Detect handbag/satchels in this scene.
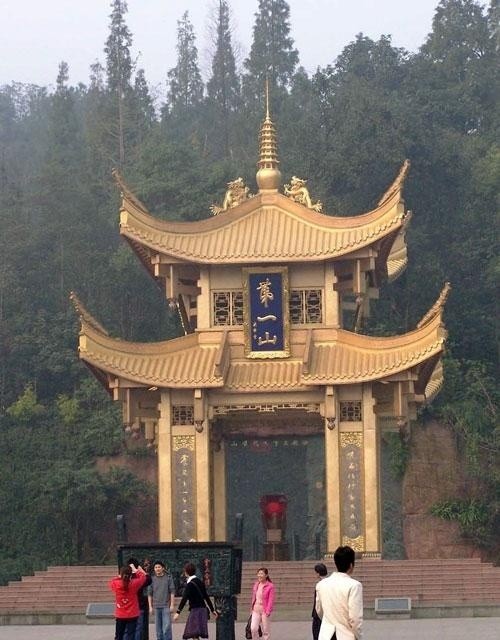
[206,607,211,620]
[245,615,262,639]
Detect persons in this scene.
[314,546,364,640]
[109,563,146,640]
[172,563,218,640]
[146,560,176,640]
[119,557,152,640]
[311,562,336,640]
[249,567,276,640]
